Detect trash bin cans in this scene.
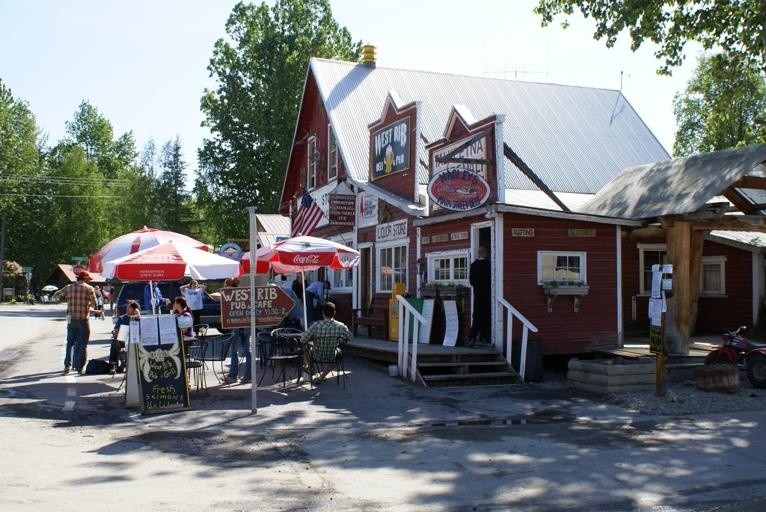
[512,340,544,383]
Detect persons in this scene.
[51,272,207,378]
[281,273,331,331]
[300,300,352,383]
[464,247,490,347]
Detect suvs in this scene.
[111,280,221,333]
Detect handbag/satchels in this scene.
[86,359,110,374]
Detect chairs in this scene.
[113,322,350,397]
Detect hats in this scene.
[79,272,93,280]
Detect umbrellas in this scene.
[88,225,210,273]
[100,240,243,314]
[240,232,362,331]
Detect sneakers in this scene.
[313,378,321,385]
[221,375,237,383]
[304,364,315,375]
[239,375,251,382]
[109,365,118,374]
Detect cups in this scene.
[385,145,394,173]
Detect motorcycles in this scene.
[704,325,766,391]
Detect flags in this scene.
[292,187,322,236]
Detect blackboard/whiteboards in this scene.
[134,327,192,416]
